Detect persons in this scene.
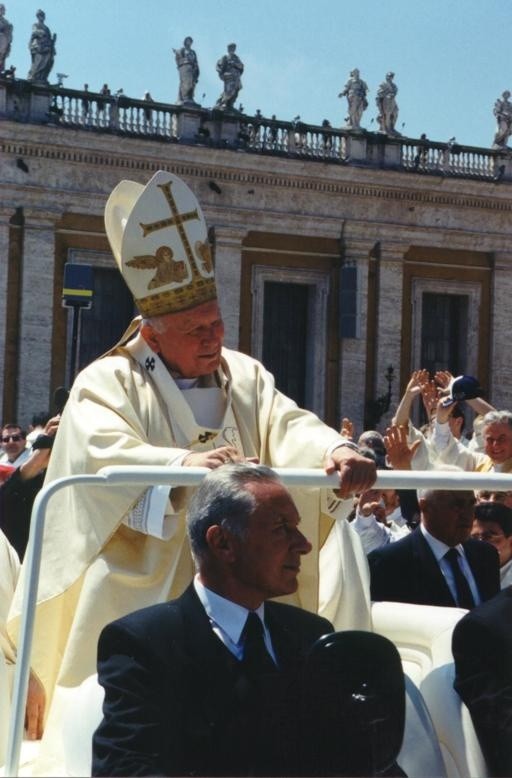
[1,4,14,72]
[142,91,156,135]
[1,365,512,611]
[416,134,430,169]
[28,9,58,82]
[375,71,400,131]
[173,36,200,103]
[2,300,379,778]
[338,68,369,126]
[95,84,111,128]
[80,83,91,122]
[492,90,512,149]
[268,114,279,150]
[449,582,511,778]
[252,109,263,142]
[58,256,378,644]
[216,42,245,108]
[87,458,414,777]
[320,119,333,157]
[0,528,54,745]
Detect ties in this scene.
[444,548,475,610]
[243,612,275,676]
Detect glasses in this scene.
[3,435,20,442]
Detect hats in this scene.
[442,375,482,407]
[104,170,217,318]
